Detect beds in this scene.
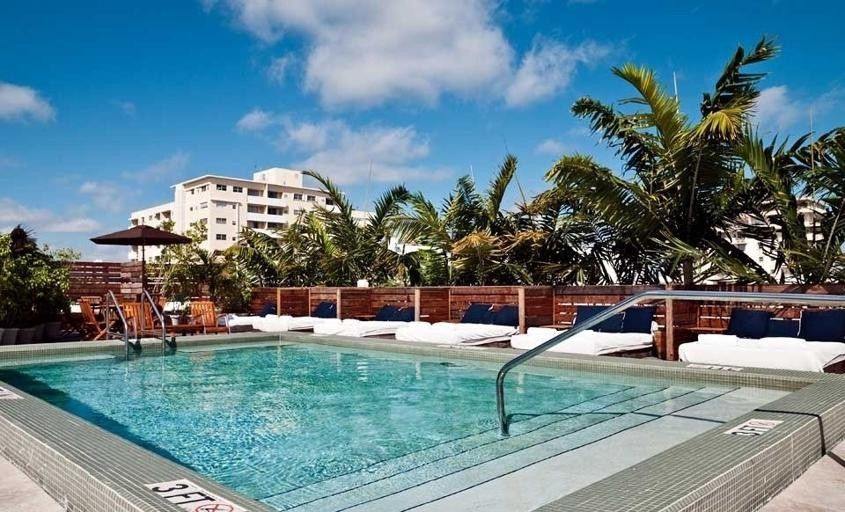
[678,335,845,374]
[511,316,658,358]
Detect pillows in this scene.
[261,304,277,317]
[573,305,657,333]
[375,304,414,322]
[311,302,336,318]
[727,308,845,341]
[460,304,519,326]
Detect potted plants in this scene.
[0,225,81,345]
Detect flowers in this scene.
[163,301,190,315]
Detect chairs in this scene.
[313,320,414,337]
[225,314,278,333]
[122,302,160,338]
[79,302,119,340]
[259,316,337,331]
[395,323,520,347]
[191,301,229,335]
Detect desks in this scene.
[155,323,204,336]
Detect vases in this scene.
[170,315,180,325]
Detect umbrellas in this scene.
[88,223,194,297]
